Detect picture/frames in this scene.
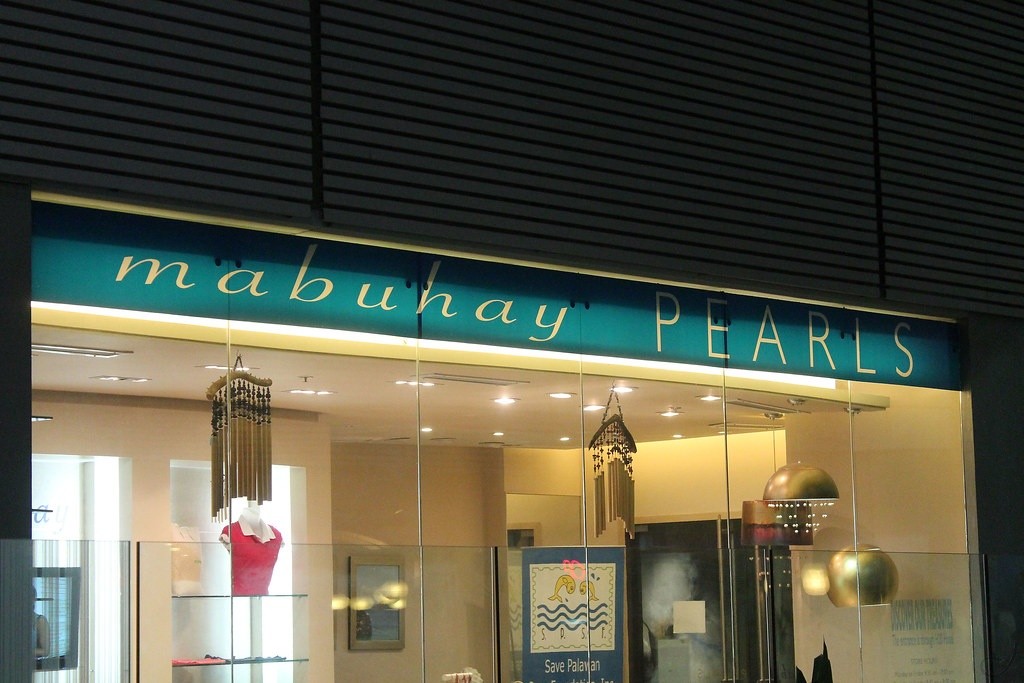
[506,523,543,557]
[350,555,405,650]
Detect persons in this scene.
[220,507,285,596]
[33,585,50,657]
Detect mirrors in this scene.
[506,492,581,683]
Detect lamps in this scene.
[740,398,899,608]
